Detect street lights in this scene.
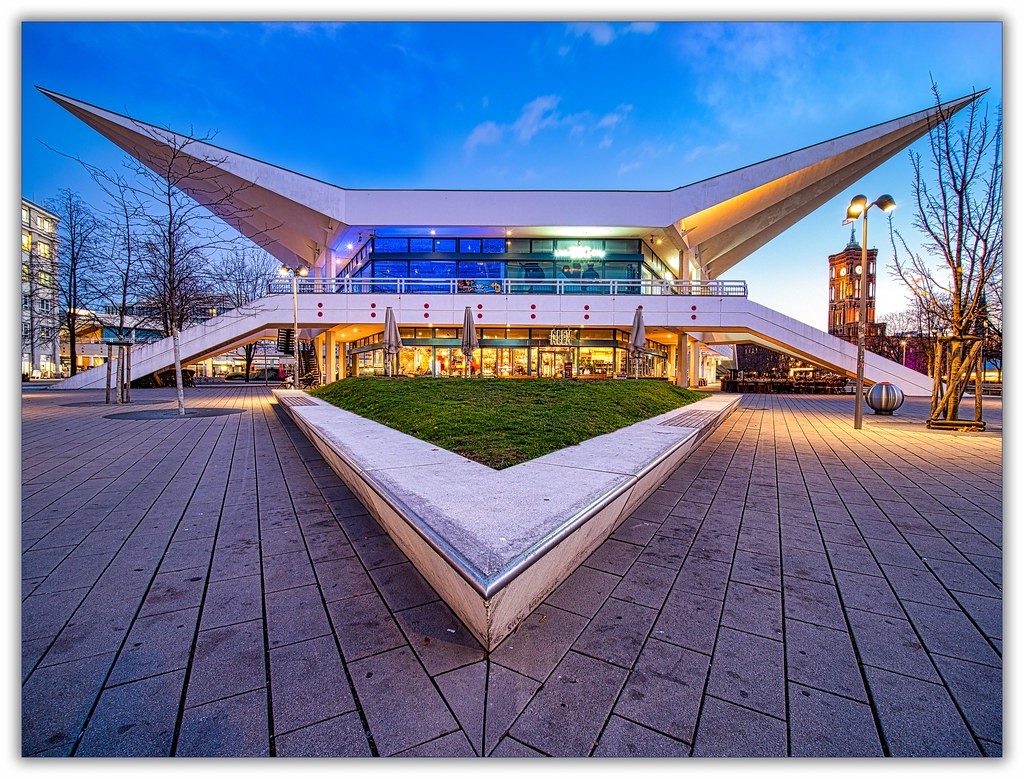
[281,264,309,390]
[846,194,897,428]
[901,341,906,366]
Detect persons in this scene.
[582,262,600,291]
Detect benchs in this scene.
[966,384,1002,396]
[277,382,294,389]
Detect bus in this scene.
[254,369,279,380]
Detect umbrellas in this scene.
[382,307,403,376]
[461,307,478,380]
[631,308,646,380]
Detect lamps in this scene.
[650,235,654,244]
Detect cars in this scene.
[225,373,246,380]
